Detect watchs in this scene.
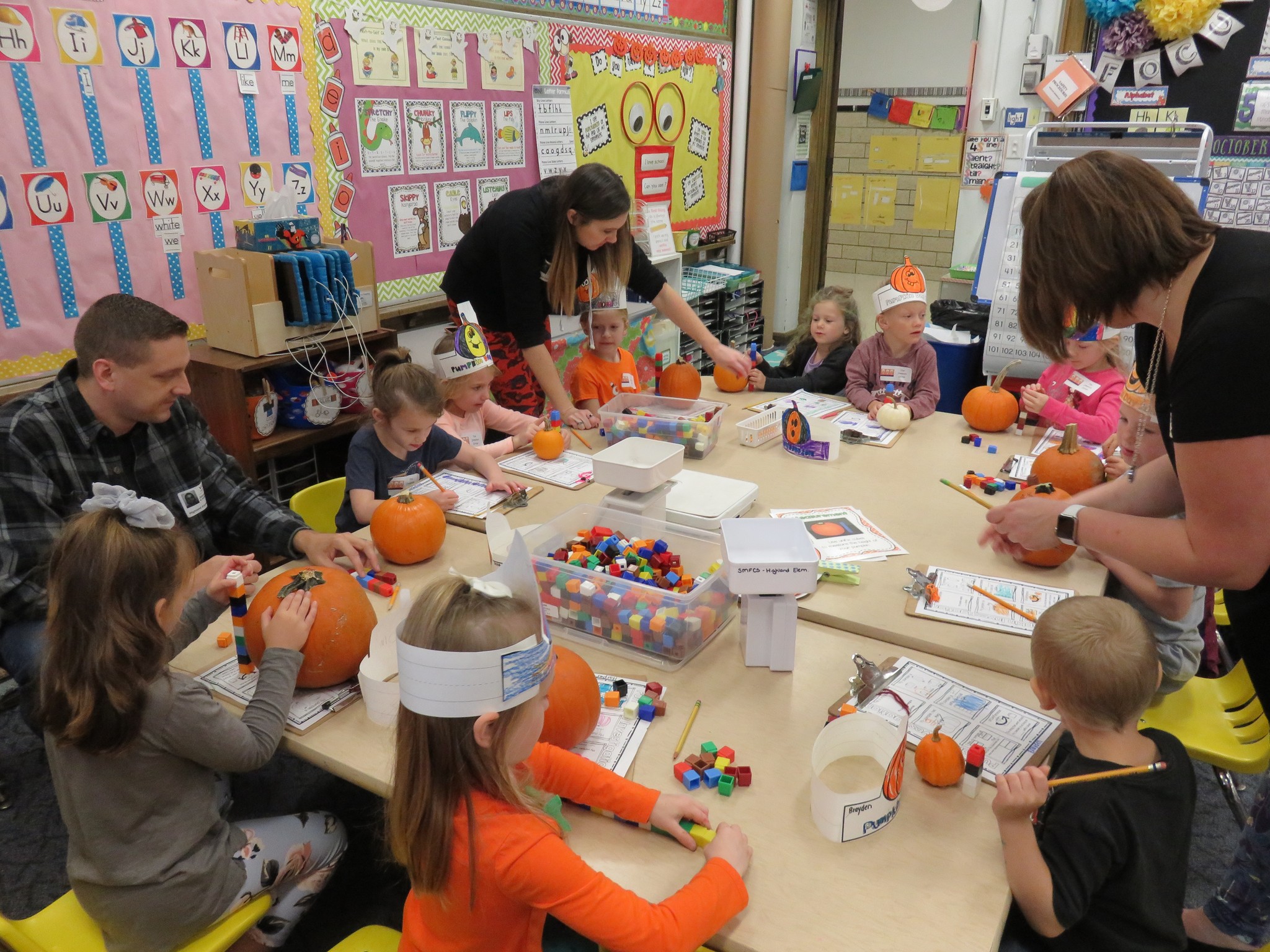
[1055,504,1086,547]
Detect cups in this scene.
[683,230,700,249]
[672,231,688,251]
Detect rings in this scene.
[577,421,583,426]
[743,354,746,358]
[588,414,593,419]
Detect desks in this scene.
[169,522,1061,952]
[391,376,1111,680]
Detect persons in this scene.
[1085,358,1219,683]
[434,330,571,472]
[0,295,380,742]
[439,163,751,430]
[745,285,862,395]
[38,482,348,952]
[1019,303,1126,444]
[992,596,1197,952]
[846,277,940,421]
[978,151,1270,952]
[334,345,527,533]
[571,309,655,420]
[389,575,752,952]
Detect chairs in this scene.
[1138,657,1269,774]
[0,890,271,952]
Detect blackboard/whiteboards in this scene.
[971,171,1211,332]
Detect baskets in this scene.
[681,277,708,302]
[707,228,738,243]
[681,266,730,294]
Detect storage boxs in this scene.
[509,504,819,672]
[592,392,728,492]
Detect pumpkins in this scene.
[370,490,447,564]
[962,358,1022,432]
[244,565,379,688]
[876,396,910,430]
[1029,422,1106,495]
[915,724,965,786]
[659,357,701,409]
[537,644,602,751]
[713,340,749,392]
[1006,482,1080,567]
[533,417,564,459]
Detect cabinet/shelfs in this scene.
[185,328,398,491]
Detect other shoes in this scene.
[1181,905,1259,952]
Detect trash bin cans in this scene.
[921,297,992,415]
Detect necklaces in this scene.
[1127,277,1175,484]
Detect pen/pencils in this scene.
[742,398,778,410]
[417,463,447,492]
[1047,762,1168,787]
[673,699,702,761]
[570,428,592,450]
[745,424,778,443]
[388,582,401,610]
[966,582,1039,623]
[937,479,994,510]
